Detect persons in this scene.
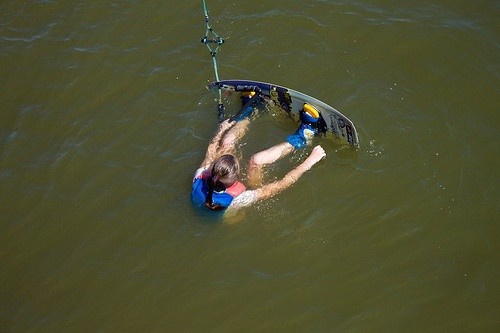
[190,95,326,225]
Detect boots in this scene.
[284,104,319,151]
[232,91,268,124]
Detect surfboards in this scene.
[206,79,359,144]
